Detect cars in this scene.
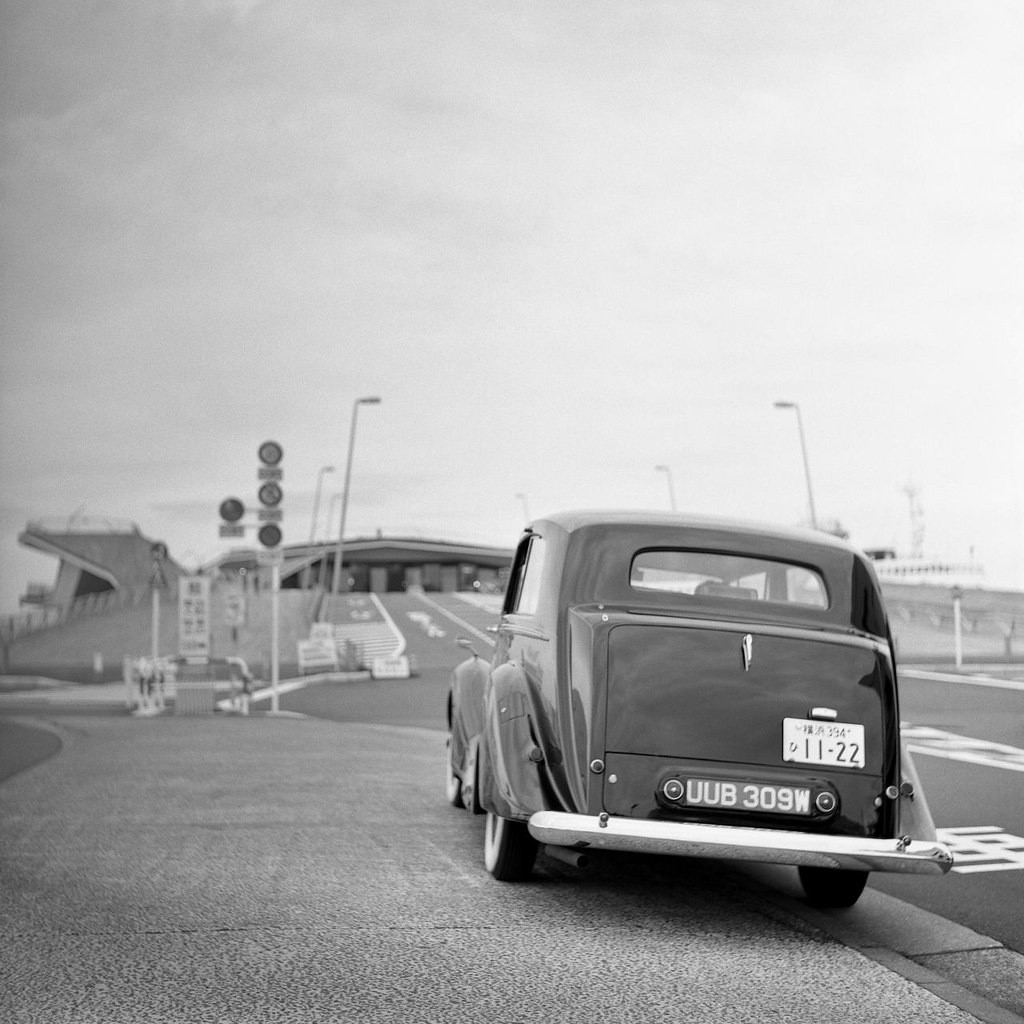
[445,506,956,913]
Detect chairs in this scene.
[700,582,757,600]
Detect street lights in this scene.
[774,398,816,532]
[655,465,675,512]
[325,393,383,641]
[298,465,336,617]
